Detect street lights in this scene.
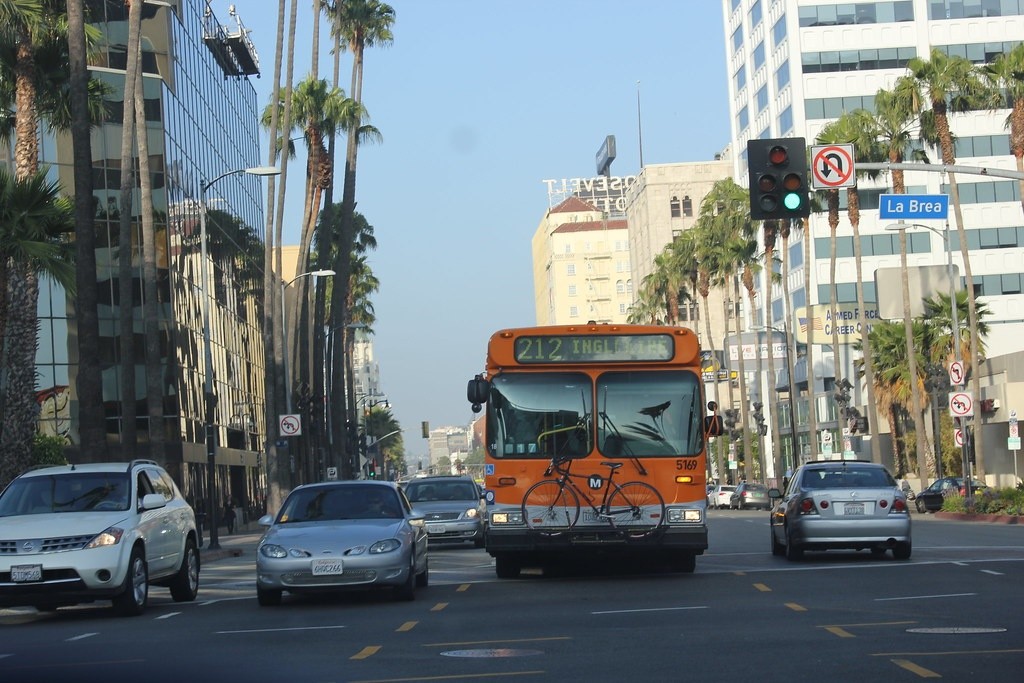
[749,324,798,470]
[923,363,946,478]
[354,392,386,474]
[886,221,972,510]
[726,410,740,486]
[279,269,338,500]
[368,399,391,480]
[834,377,869,460]
[204,166,283,548]
[753,402,768,486]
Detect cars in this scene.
[767,460,912,562]
[730,482,772,512]
[406,476,486,547]
[915,476,992,514]
[707,485,715,495]
[707,482,738,510]
[255,479,428,603]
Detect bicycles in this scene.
[521,455,665,539]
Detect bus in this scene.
[467,321,725,578]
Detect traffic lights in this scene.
[457,461,461,471]
[370,464,374,477]
[746,136,809,220]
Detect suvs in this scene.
[0,458,203,613]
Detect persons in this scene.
[449,486,469,499]
[223,508,236,534]
[101,479,143,509]
[707,476,747,485]
[418,486,438,501]
[362,493,389,514]
